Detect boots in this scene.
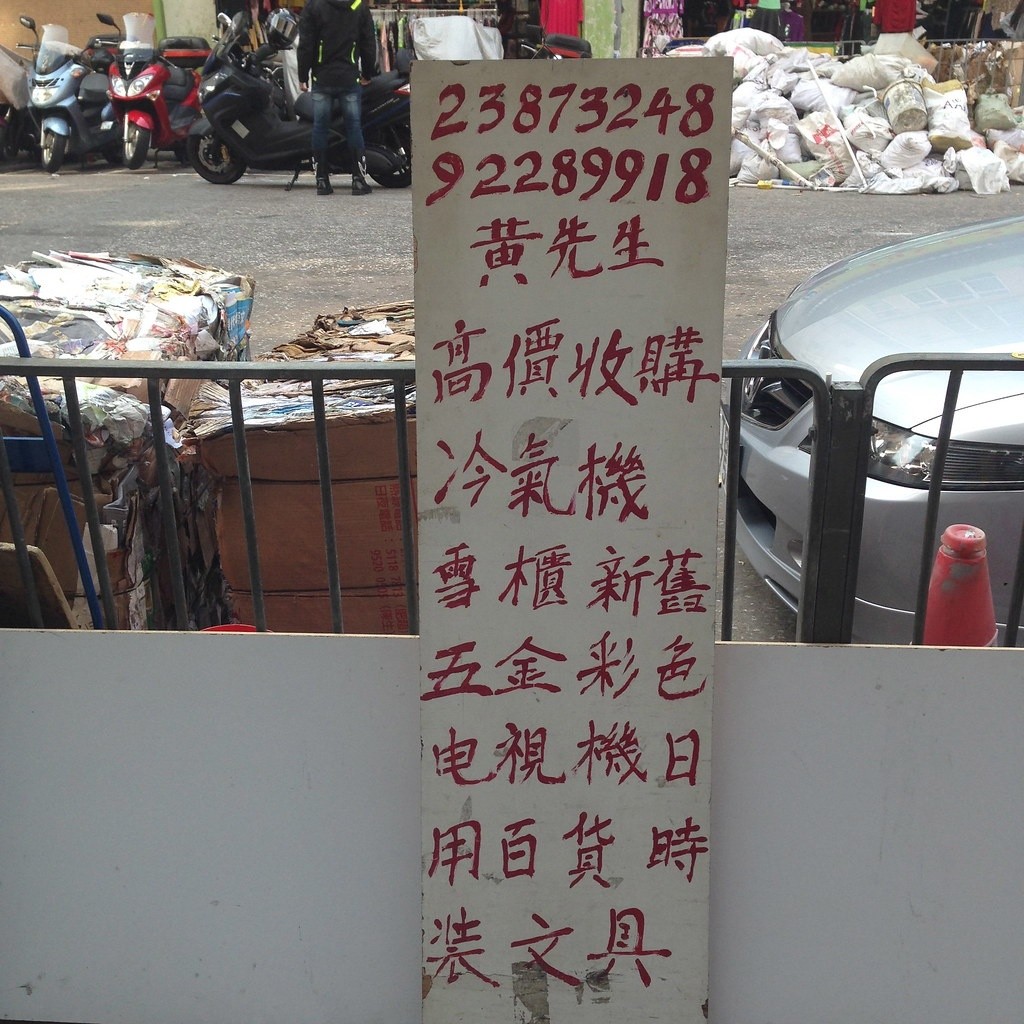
[348,148,371,194]
[312,147,335,195]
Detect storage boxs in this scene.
[162,38,211,71]
[86,32,121,65]
[204,412,418,635]
[542,34,594,59]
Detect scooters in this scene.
[519,23,595,59]
[180,9,414,192]
[0,12,214,175]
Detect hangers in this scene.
[782,2,792,12]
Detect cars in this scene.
[722,214,1024,647]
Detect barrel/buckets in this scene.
[882,77,927,134]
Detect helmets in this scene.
[91,48,116,73]
[266,8,299,48]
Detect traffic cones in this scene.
[929,525,1002,653]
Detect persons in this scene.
[495,0,515,59]
[297,0,377,196]
[231,0,268,31]
[262,0,300,100]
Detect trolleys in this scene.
[1,304,104,630]
